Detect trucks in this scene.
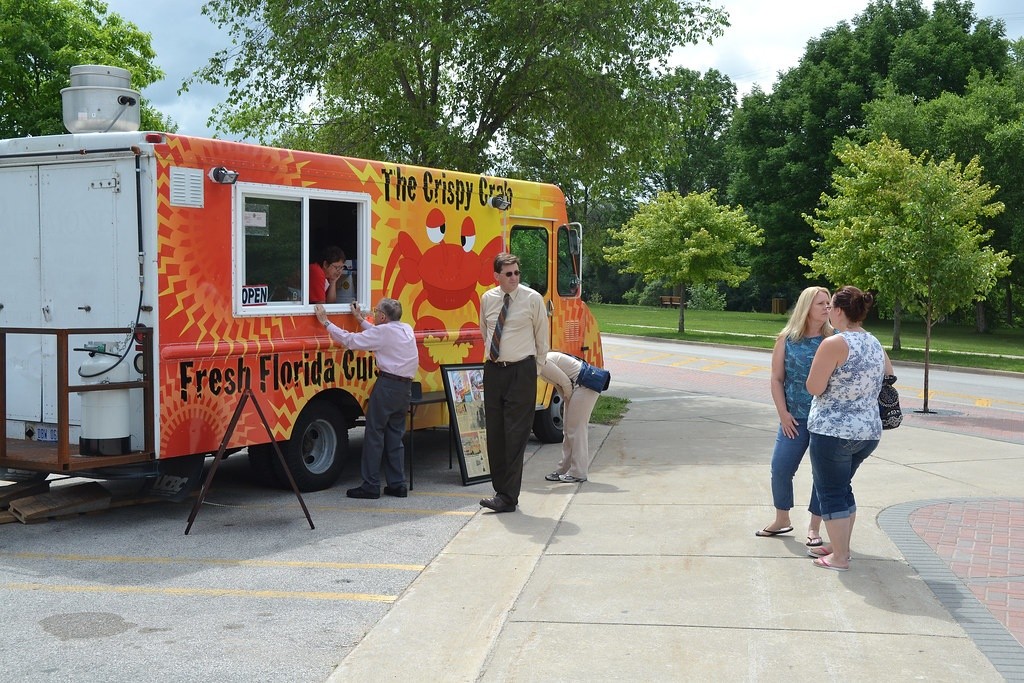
[0,130,605,494]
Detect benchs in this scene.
[670,297,688,310]
[660,295,678,308]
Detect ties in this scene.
[490,295,510,363]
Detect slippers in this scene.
[805,536,823,546]
[812,556,850,572]
[755,524,793,536]
[807,547,852,560]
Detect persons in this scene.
[805,285,895,572]
[314,297,419,499]
[534,347,601,483]
[755,286,840,546]
[478,252,550,510]
[294,244,346,303]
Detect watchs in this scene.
[324,322,329,326]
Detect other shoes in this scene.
[479,495,516,513]
[383,485,410,498]
[347,486,380,499]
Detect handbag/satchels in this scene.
[878,346,904,429]
[575,360,611,393]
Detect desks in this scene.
[407,390,452,491]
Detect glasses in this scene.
[332,262,346,272]
[826,303,844,314]
[500,269,521,277]
[372,307,387,319]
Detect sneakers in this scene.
[558,473,588,482]
[545,472,560,481]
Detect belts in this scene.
[485,357,532,368]
